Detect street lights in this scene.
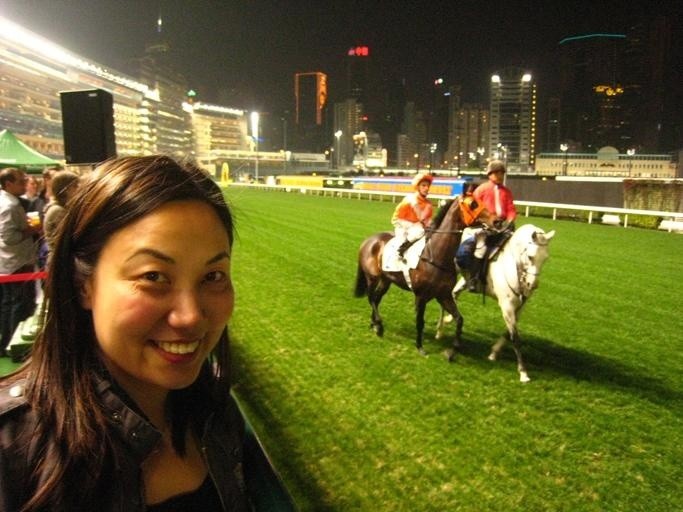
[496,144,509,189]
[360,132,368,169]
[625,147,635,178]
[559,143,567,176]
[334,129,341,168]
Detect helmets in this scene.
[486,160,505,176]
[412,173,433,189]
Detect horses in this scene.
[435,221,557,385]
[352,182,506,362]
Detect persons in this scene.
[0,165,80,359]
[0,149,237,511]
[388,173,435,266]
[467,160,517,292]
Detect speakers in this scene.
[58,88,117,166]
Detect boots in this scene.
[463,271,476,291]
[396,237,412,259]
